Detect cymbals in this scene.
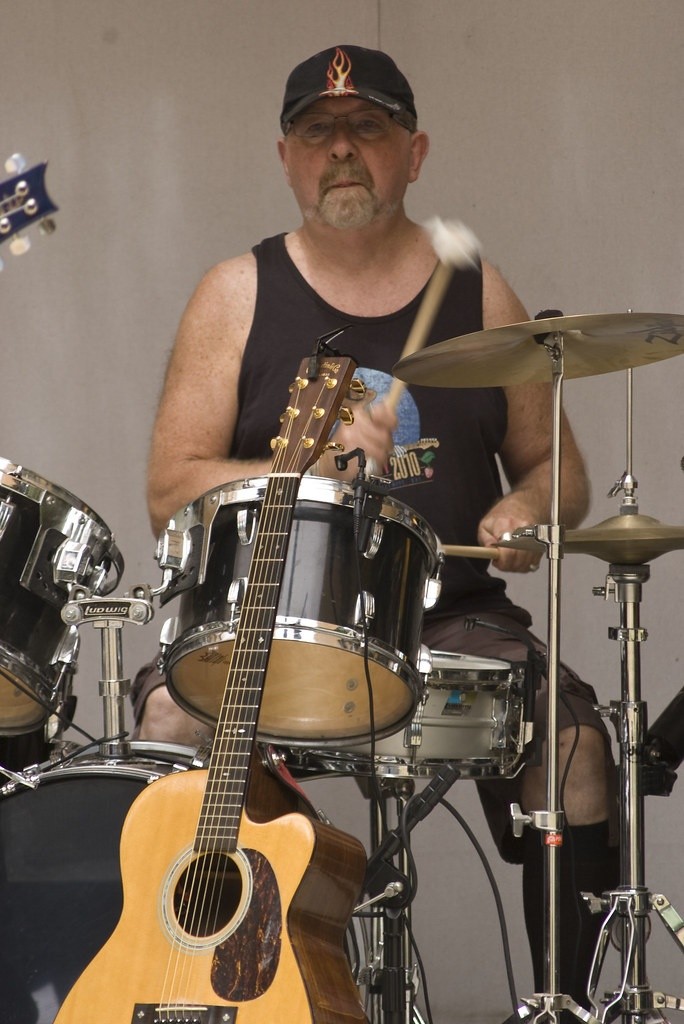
[392,310,684,389]
[491,514,684,564]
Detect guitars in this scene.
[55,348,369,1022]
[0,153,57,267]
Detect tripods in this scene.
[494,333,683,1024]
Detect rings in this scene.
[530,564,539,571]
[503,532,513,541]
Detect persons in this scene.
[130,44,623,1024]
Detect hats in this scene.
[280,45,418,131]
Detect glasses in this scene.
[284,107,413,138]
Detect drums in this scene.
[273,647,528,779]
[162,473,445,748]
[0,459,118,740]
[0,738,369,1024]
